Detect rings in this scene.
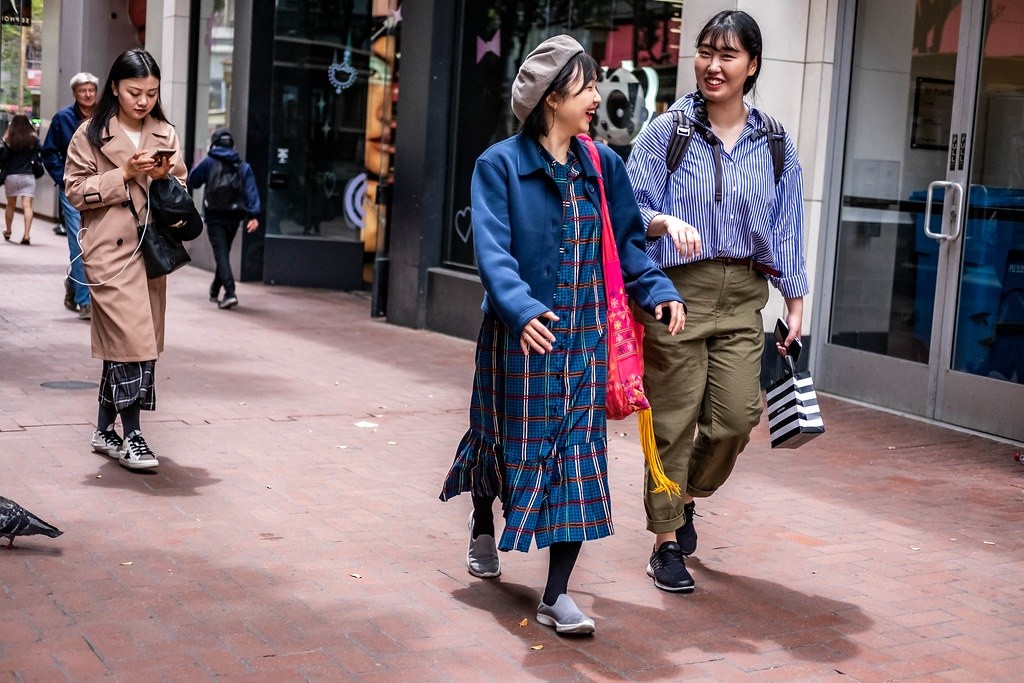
[523,334,529,340]
[682,313,686,317]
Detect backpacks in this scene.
[210,154,246,218]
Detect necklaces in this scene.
[558,156,567,163]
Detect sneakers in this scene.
[92,430,124,459]
[120,431,159,468]
[678,502,696,556]
[647,540,695,591]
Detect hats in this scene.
[511,34,583,123]
[70,71,101,86]
[212,128,235,148]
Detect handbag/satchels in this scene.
[766,355,826,449]
[137,223,192,278]
[32,156,45,178]
[148,173,204,240]
[606,302,650,421]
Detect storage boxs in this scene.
[910,186,1024,376]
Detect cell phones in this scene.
[774,318,802,364]
[149,149,176,167]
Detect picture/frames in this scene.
[911,77,954,150]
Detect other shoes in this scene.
[468,512,501,578]
[536,594,595,634]
[54,227,67,235]
[220,294,238,309]
[211,293,218,300]
[79,305,93,319]
[20,237,31,244]
[4,231,11,240]
[64,278,77,311]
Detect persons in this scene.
[1,112,42,245]
[626,10,809,591]
[439,34,688,633]
[187,129,260,310]
[64,49,187,469]
[303,171,325,235]
[42,71,99,318]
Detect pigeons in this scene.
[0,496,64,551]
